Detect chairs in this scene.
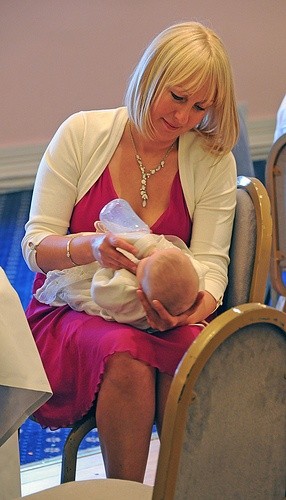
[21,303,286,500]
[265,133,285,312]
[60,176,272,483]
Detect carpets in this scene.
[1,160,286,473]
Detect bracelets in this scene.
[66,232,81,267]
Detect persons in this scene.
[22,22,237,484]
[45,232,210,330]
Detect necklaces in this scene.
[128,118,177,208]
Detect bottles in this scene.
[99,199,152,246]
